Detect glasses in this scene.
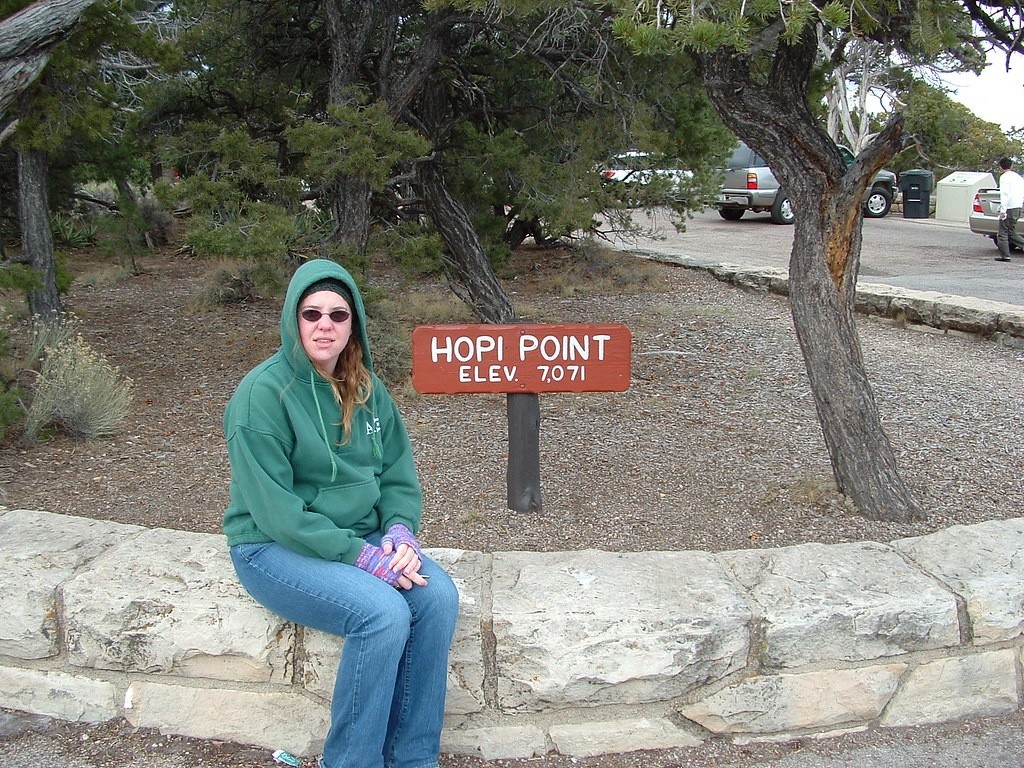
[298,310,353,322]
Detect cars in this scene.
[590,151,698,208]
[969,188,1024,251]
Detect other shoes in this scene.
[995,257,1010,262]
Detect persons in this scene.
[143,167,195,218]
[222,259,459,768]
[995,156,1024,262]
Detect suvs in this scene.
[702,140,899,225]
